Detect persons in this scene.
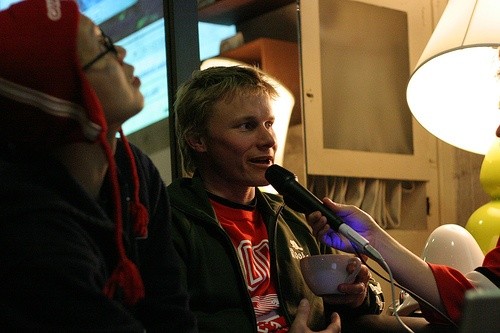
[0,0,198,333]
[166,66,385,333]
[305,197,500,333]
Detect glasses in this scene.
[82,29,118,72]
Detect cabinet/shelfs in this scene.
[162,0,443,318]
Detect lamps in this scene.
[406,0,500,156]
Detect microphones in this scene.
[264,165,369,250]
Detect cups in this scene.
[300,255,362,296]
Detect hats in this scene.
[0,0,148,303]
